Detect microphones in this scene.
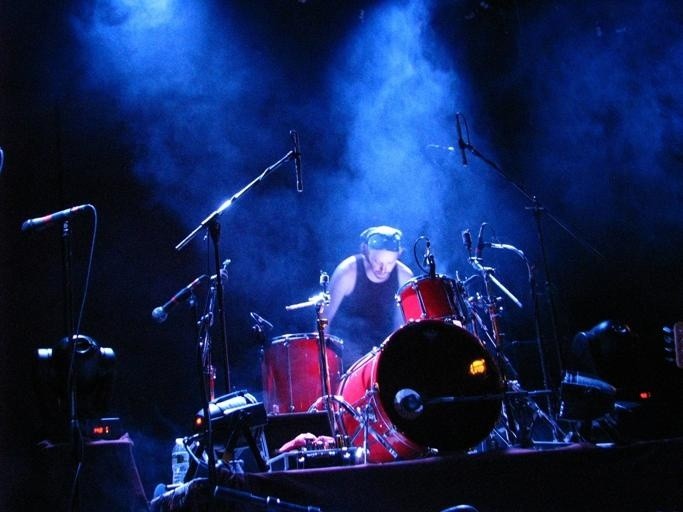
[252,313,274,330]
[21,204,89,236]
[293,133,303,192]
[459,274,480,295]
[475,226,483,261]
[423,241,430,273]
[455,114,469,165]
[462,229,473,247]
[403,392,423,412]
[150,274,208,324]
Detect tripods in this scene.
[461,257,579,443]
[305,299,402,462]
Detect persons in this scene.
[318,225,414,373]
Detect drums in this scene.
[395,273,469,327]
[333,320,503,462]
[259,332,344,412]
